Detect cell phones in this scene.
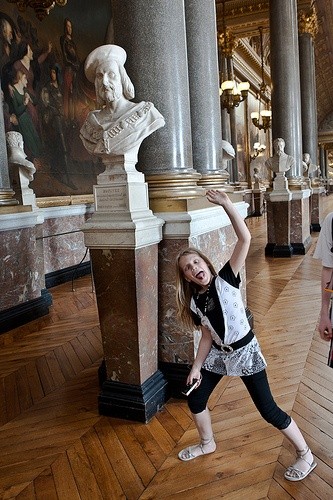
[182,379,199,396]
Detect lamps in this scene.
[250,27,272,133]
[216,0,250,114]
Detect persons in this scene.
[6,132,36,182]
[265,138,293,173]
[303,153,317,173]
[313,211,333,370]
[79,45,165,156]
[176,190,317,481]
